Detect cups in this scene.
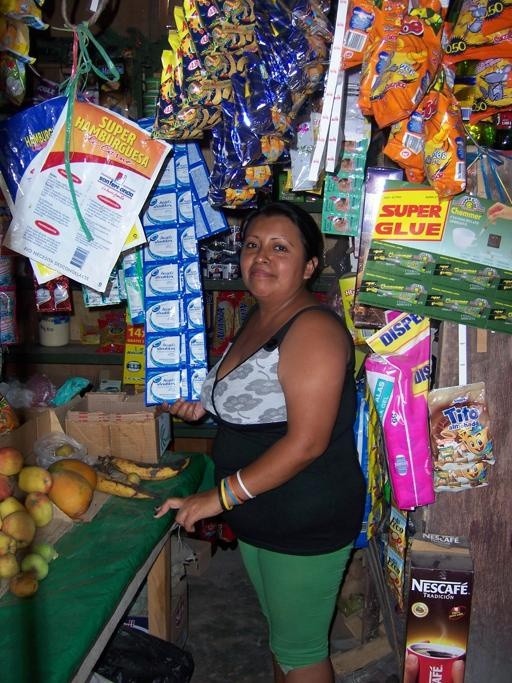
[408,643,464,683]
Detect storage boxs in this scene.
[0,390,172,500]
[172,535,213,577]
[120,578,189,651]
[309,213,339,273]
[69,290,113,344]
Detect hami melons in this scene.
[48,459,97,491]
[48,469,92,518]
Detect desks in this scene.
[1,340,135,396]
[167,421,219,455]
[0,447,217,682]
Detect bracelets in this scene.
[216,468,256,514]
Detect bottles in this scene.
[37,314,71,347]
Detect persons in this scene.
[152,201,364,682]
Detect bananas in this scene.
[97,476,158,498]
[108,456,191,481]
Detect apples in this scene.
[0,447,53,598]
